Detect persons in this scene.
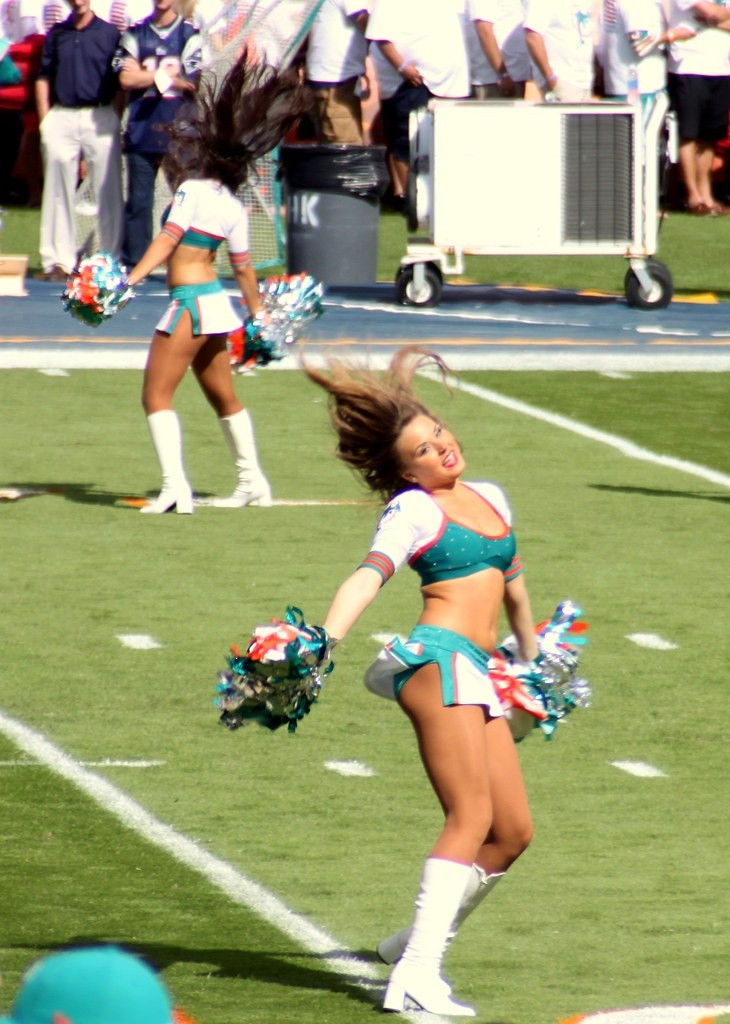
[364,0,472,213]
[303,0,372,148]
[112,0,203,285]
[467,0,532,101]
[313,408,542,1017]
[601,1,696,222]
[666,0,730,218]
[122,151,270,516]
[522,0,602,101]
[34,0,127,282]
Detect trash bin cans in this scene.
[274,141,391,288]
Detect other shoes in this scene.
[41,265,69,282]
[689,200,726,221]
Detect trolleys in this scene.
[396,91,676,312]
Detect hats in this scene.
[3,946,172,1022]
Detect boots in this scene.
[213,408,273,508]
[380,857,506,1018]
[140,411,193,515]
